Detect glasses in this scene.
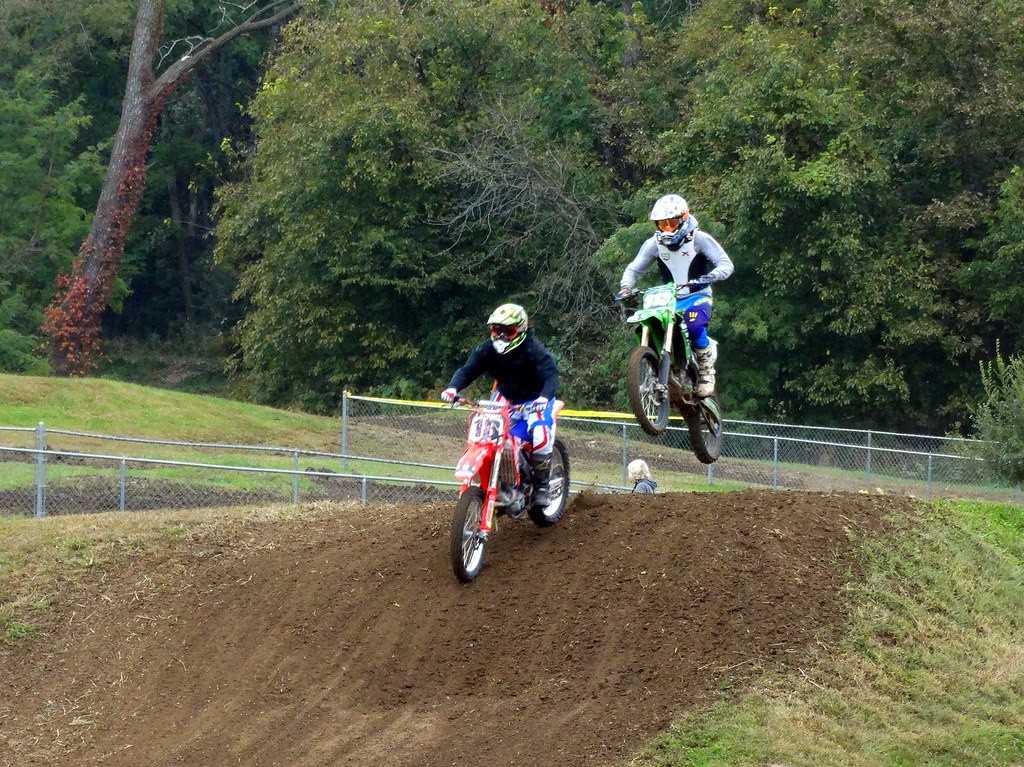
[658,220,680,233]
[491,325,518,339]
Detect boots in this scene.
[532,459,552,508]
[695,342,716,395]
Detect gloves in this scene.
[441,388,456,403]
[699,275,712,284]
[618,289,628,298]
[530,397,548,412]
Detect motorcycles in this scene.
[617,277,724,464]
[442,392,571,581]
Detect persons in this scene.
[441,303,559,508]
[628,460,657,494]
[618,194,734,397]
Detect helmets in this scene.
[649,193,699,245]
[486,303,528,354]
